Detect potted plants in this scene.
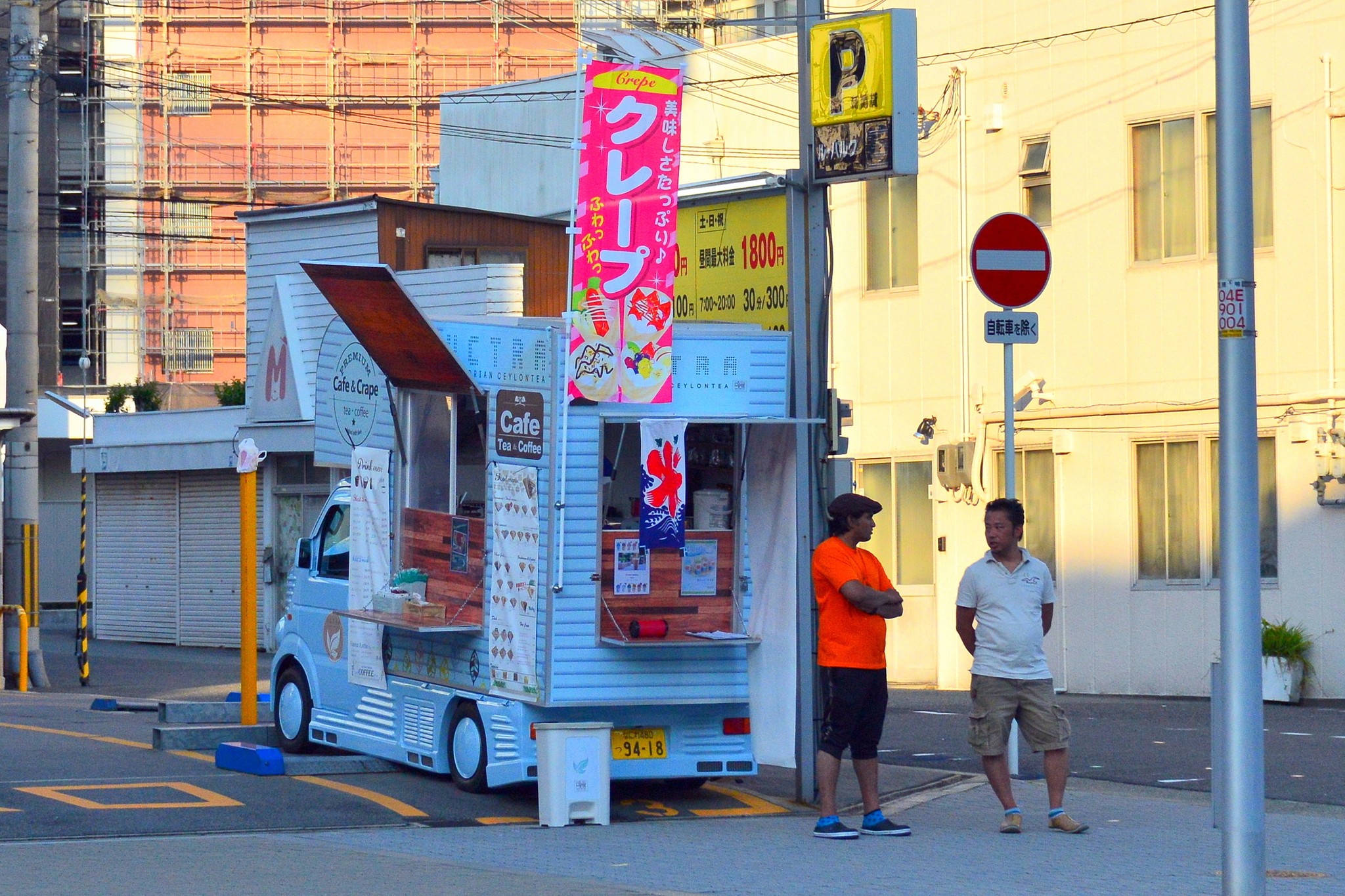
[1261,614,1337,704]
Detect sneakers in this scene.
[1000,813,1023,833]
[1048,813,1089,834]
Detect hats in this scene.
[827,492,883,514]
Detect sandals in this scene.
[860,817,912,837]
[813,822,859,840]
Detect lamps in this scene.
[913,415,937,440]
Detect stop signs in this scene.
[969,210,1054,306]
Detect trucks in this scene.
[270,310,799,791]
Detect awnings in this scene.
[602,419,828,528]
[299,259,487,464]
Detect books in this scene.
[685,630,748,640]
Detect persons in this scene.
[811,492,913,839]
[955,498,1089,835]
